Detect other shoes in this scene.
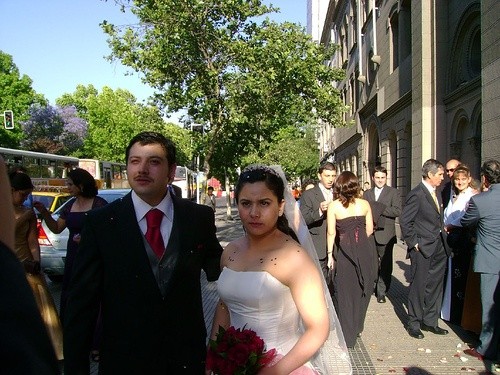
[464,349,486,357]
[375,293,386,303]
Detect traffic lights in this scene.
[4,110,14,129]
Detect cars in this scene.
[23,188,132,283]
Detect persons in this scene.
[399,158,449,339]
[324,171,376,350]
[202,186,216,216]
[300,161,340,301]
[62,130,224,375]
[361,166,402,303]
[288,177,318,242]
[443,156,500,365]
[359,181,372,198]
[0,151,115,375]
[202,165,332,375]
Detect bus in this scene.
[0,147,80,193]
[172,165,208,199]
[61,158,131,189]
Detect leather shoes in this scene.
[421,323,449,335]
[404,324,425,339]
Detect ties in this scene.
[432,192,440,214]
[325,190,331,206]
[143,207,165,258]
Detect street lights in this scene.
[183,106,193,169]
